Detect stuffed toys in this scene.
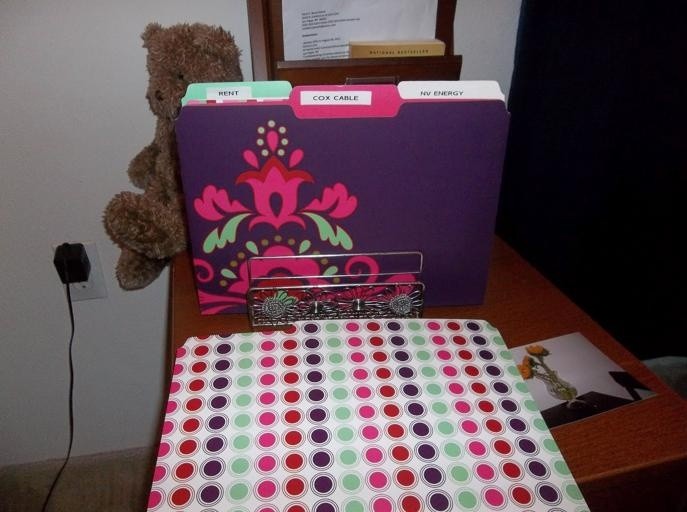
[101,21,245,291]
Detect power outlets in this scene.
[52,242,107,304]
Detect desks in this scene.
[167,231,685,511]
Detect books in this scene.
[347,39,446,58]
